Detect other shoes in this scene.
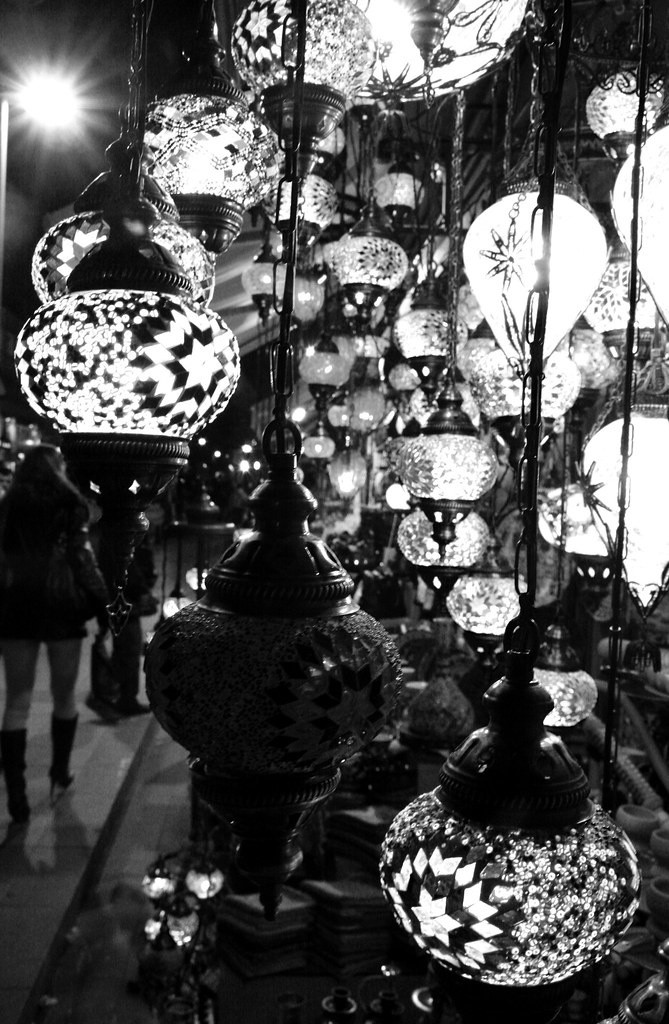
[115,697,148,714]
[85,693,121,722]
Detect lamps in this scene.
[15,0,669,1024]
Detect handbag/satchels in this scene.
[47,530,109,620]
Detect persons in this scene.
[86,532,160,720]
[0,444,110,823]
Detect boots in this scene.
[1,729,29,821]
[48,713,78,799]
